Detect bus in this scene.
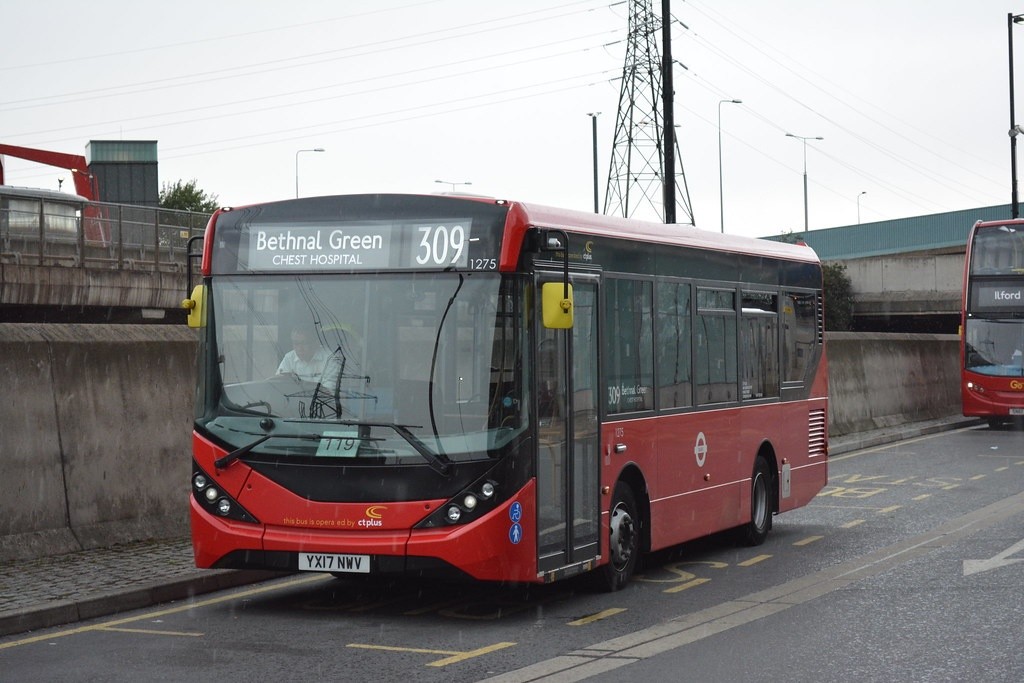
[179,192,832,594]
[957,218,1024,431]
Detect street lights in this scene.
[717,99,743,234]
[71,167,95,201]
[857,191,867,224]
[587,111,601,214]
[784,133,824,231]
[434,180,473,193]
[1005,12,1024,219]
[295,147,327,198]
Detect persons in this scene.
[274,321,343,419]
